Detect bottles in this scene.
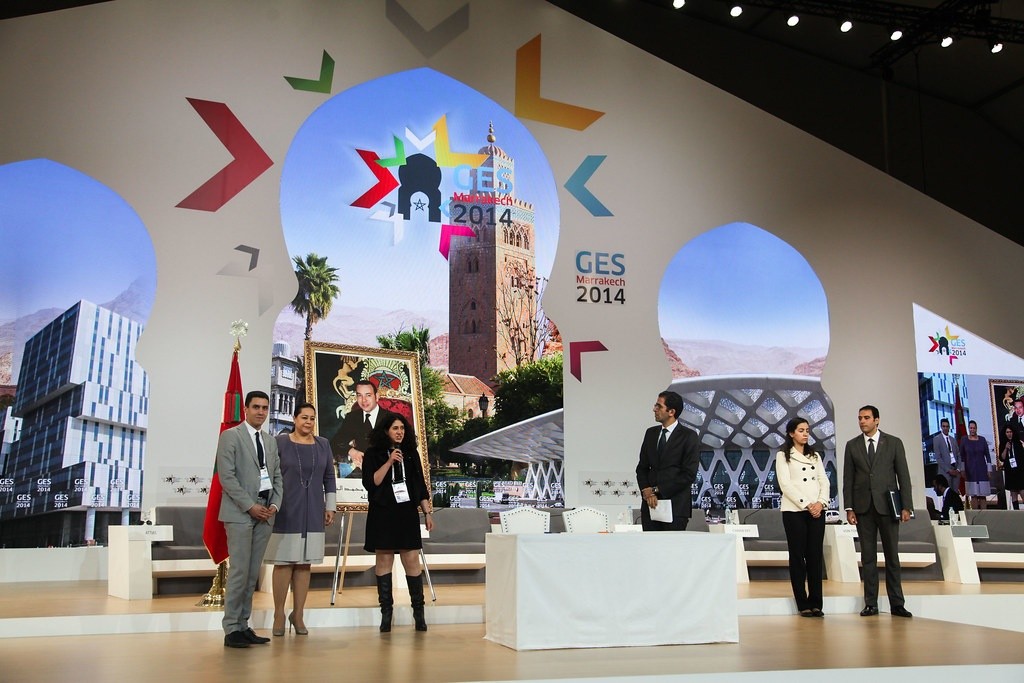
[725,506,731,524]
[948,507,954,525]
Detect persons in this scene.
[932,474,963,525]
[934,399,1024,510]
[776,417,830,617]
[842,405,913,618]
[636,390,701,533]
[331,380,392,479]
[263,402,336,636]
[217,391,284,647]
[362,412,435,631]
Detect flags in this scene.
[202,350,245,565]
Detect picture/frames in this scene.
[988,378,1024,470]
[304,338,433,513]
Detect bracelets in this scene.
[424,511,430,516]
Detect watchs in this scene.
[652,487,657,494]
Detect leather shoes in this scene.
[890,606,912,617]
[860,605,879,616]
[800,608,823,617]
[224,628,270,648]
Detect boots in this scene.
[405,573,427,632]
[375,572,393,632]
[1012,500,1019,511]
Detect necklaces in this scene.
[292,434,315,488]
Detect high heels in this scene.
[288,611,308,634]
[272,613,286,636]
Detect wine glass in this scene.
[951,514,958,525]
[729,513,736,525]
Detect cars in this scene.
[824,510,843,524]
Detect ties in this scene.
[1009,442,1013,459]
[254,432,269,500]
[363,413,372,435]
[943,496,945,505]
[946,437,951,454]
[1020,418,1022,427]
[868,439,875,466]
[658,429,669,461]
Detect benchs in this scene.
[636,506,1024,554]
[148,507,491,559]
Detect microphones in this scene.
[394,442,401,466]
[743,510,759,524]
[972,490,1001,525]
